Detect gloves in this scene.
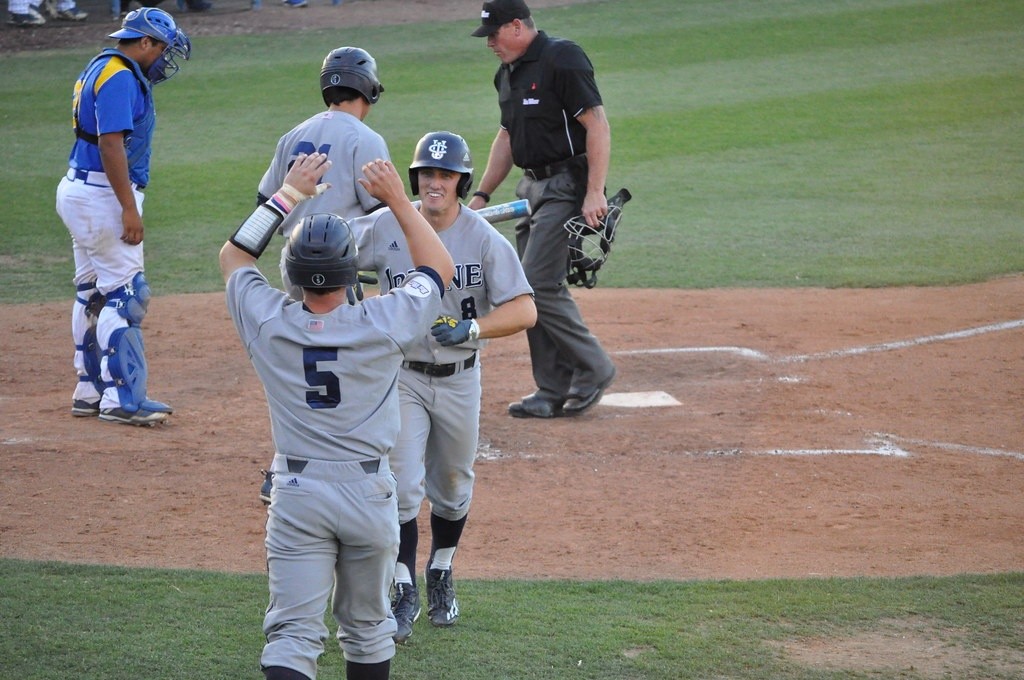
[431,314,479,347]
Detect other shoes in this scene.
[285,0,307,7]
[260,469,272,503]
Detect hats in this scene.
[472,0,530,39]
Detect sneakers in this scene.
[424,561,459,627]
[388,580,423,643]
[72,395,100,417]
[8,10,47,25]
[98,403,168,428]
[56,7,89,20]
[560,367,616,414]
[508,402,532,418]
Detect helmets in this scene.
[108,7,176,45]
[407,131,474,200]
[320,46,385,104]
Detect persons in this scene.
[355,133,539,644]
[257,45,395,504]
[219,150,455,680]
[57,7,178,427]
[7,0,342,26]
[466,0,616,419]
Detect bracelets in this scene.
[472,192,490,202]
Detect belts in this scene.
[287,459,380,476]
[402,356,478,378]
[75,170,88,180]
[525,166,570,182]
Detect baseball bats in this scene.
[475,199,532,225]
